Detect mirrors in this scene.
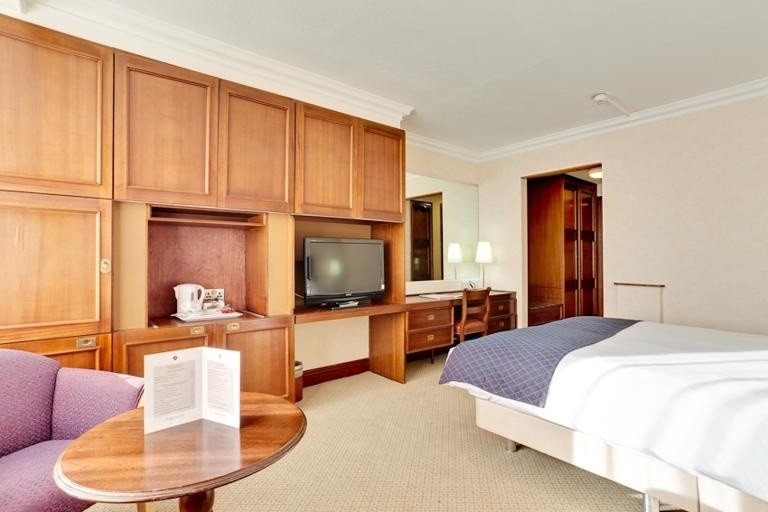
[405,172,479,281]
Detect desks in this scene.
[53,391,307,512]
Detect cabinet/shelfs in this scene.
[287,216,407,402]
[410,199,434,280]
[114,50,220,211]
[112,317,296,402]
[112,203,286,328]
[358,117,407,222]
[2,334,111,373]
[0,191,114,341]
[1,15,114,200]
[294,100,358,219]
[407,289,518,364]
[219,79,296,214]
[528,174,598,319]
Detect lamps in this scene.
[447,243,463,280]
[475,243,493,289]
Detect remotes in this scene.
[337,301,359,308]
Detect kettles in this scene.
[173,283,205,313]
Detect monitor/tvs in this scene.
[303,236,386,310]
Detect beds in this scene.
[438,315,768,512]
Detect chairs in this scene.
[0,350,146,512]
[455,287,491,343]
